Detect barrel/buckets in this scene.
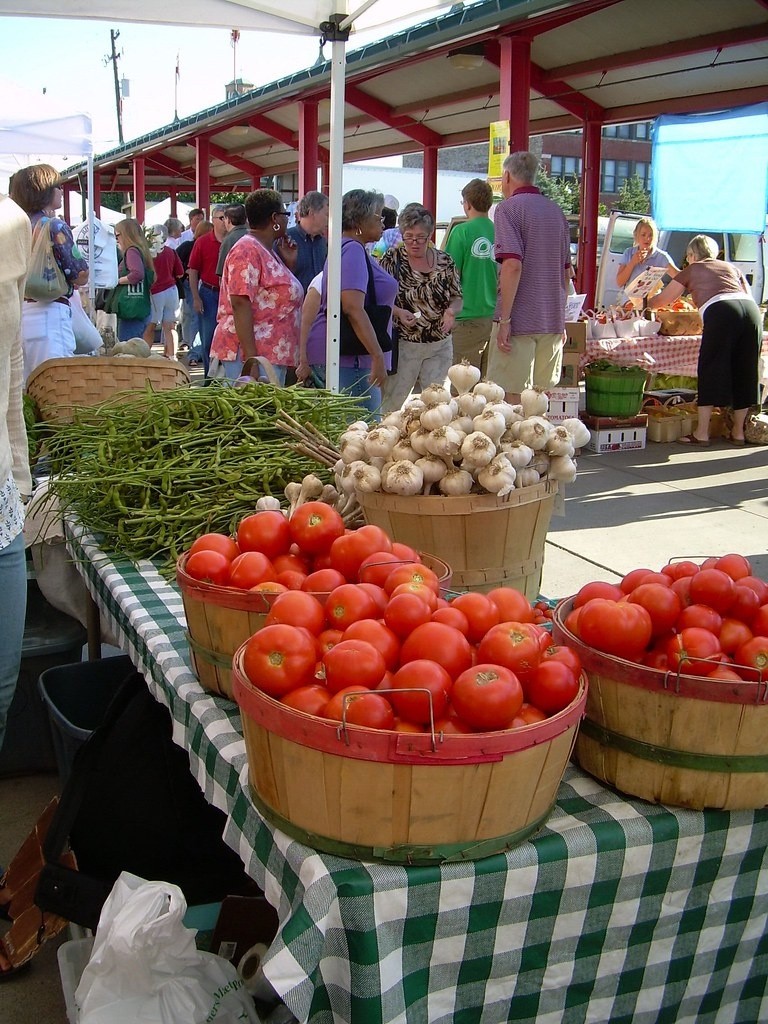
[549,589,768,811]
[231,616,594,862]
[346,480,555,609]
[585,368,647,417]
[39,654,138,779]
[173,543,453,709]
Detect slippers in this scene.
[676,433,709,447]
[723,432,745,445]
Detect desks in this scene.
[61,510,768,1024]
[577,335,702,380]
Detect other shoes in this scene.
[180,345,187,350]
[189,360,197,365]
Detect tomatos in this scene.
[563,553,768,683]
[183,502,583,735]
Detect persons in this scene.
[486,151,570,405]
[0,193,33,754]
[647,234,763,447]
[9,164,90,395]
[379,203,463,417]
[616,219,683,306]
[94,178,399,426]
[443,178,497,398]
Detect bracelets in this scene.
[499,318,511,324]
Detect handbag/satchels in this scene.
[68,289,104,354]
[104,246,152,320]
[22,217,69,302]
[324,240,392,357]
[578,303,663,340]
[75,871,262,1024]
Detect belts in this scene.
[202,282,219,292]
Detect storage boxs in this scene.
[556,353,580,388]
[579,410,649,453]
[57,936,100,1024]
[563,321,586,353]
[37,652,132,788]
[0,579,87,778]
[642,405,722,442]
[545,387,578,424]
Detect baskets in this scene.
[25,356,192,434]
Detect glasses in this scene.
[374,214,386,223]
[116,233,121,240]
[271,210,291,218]
[402,232,431,245]
[214,215,224,221]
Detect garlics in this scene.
[257,358,591,512]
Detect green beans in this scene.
[68,379,344,576]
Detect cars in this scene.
[565,207,768,315]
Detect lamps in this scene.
[116,164,129,174]
[229,122,249,136]
[446,43,486,72]
[170,143,188,153]
